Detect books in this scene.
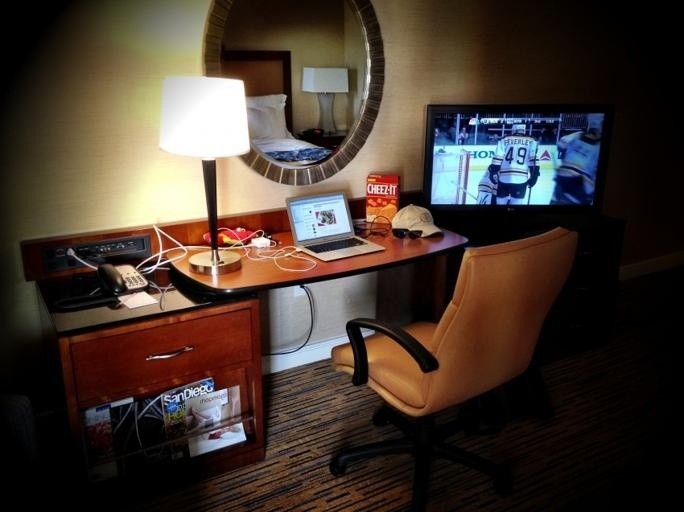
[84,396,135,458]
[161,377,215,461]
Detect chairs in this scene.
[328,225,581,512]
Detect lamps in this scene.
[157,73,251,276]
[301,65,352,134]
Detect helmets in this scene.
[587,114,604,133]
[510,122,527,137]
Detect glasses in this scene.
[392,228,423,239]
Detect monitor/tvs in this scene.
[422,99,610,226]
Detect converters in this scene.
[251,236,271,248]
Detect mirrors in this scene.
[200,0,386,188]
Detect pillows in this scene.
[245,94,292,139]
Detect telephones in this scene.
[101,263,149,297]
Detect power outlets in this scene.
[40,234,155,274]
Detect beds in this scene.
[252,138,333,171]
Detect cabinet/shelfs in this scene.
[36,284,267,500]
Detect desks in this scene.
[165,216,470,337]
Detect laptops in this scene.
[286,188,386,262]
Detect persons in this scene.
[183,406,212,436]
[478,124,540,206]
[458,128,469,144]
[550,113,605,206]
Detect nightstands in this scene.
[297,131,347,153]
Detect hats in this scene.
[390,203,444,242]
